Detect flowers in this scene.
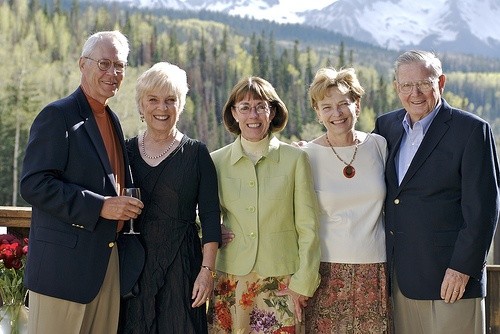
[0,234,36,270]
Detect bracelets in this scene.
[200,266,218,279]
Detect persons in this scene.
[290,50,500,334]
[118,62,221,334]
[195,77,321,334]
[20,31,144,334]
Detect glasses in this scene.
[397,80,439,94]
[234,104,272,114]
[84,56,128,73]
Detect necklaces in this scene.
[142,129,179,159]
[326,130,362,178]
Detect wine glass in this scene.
[122,188,142,235]
